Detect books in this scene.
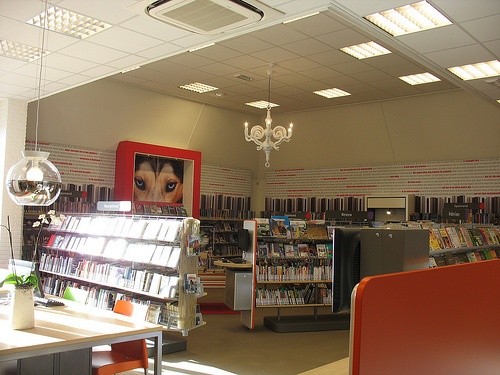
[16,179,372,331]
[385,194,500,270]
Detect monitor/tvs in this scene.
[238,228,250,252]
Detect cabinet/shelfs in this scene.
[23,210,500,331]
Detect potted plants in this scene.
[0,273,38,329]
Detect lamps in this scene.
[5,0,62,206]
[244,69,293,168]
[385,209,392,215]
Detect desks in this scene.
[0,293,164,375]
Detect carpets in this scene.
[200,303,240,314]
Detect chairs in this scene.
[91,300,148,375]
[63,286,89,306]
[349,259,500,375]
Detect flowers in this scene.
[30,209,67,298]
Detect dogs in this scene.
[134,154,184,202]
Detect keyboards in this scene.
[229,258,244,263]
[33,295,64,306]
